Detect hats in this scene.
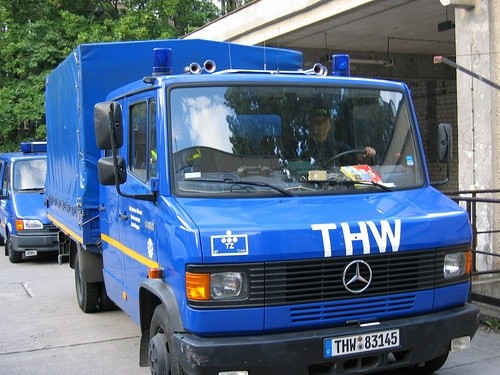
[309,106,331,118]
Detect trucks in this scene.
[42,37,480,375]
[0,141,60,263]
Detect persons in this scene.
[295,104,376,166]
